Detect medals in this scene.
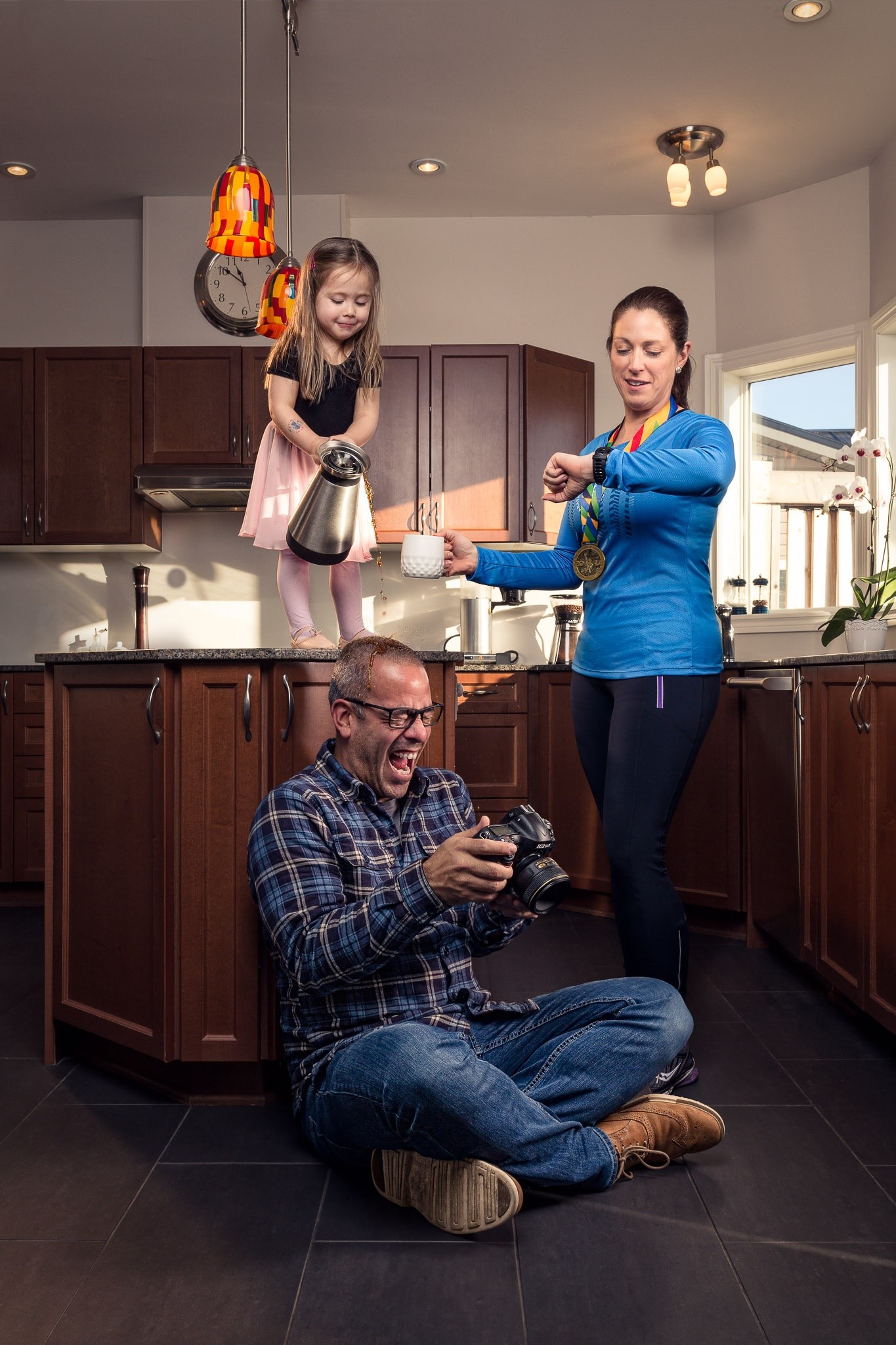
[573,540,606,581]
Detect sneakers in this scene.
[648,1042,699,1095]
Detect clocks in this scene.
[193,243,288,338]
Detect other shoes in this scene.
[291,627,338,650]
[338,628,367,650]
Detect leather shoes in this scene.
[598,1094,726,1186]
[370,1149,524,1235]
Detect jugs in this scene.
[285,438,372,567]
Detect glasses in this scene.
[343,698,445,729]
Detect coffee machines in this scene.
[443,575,531,665]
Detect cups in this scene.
[400,534,447,579]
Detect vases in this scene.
[844,619,888,653]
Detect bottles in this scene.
[729,574,749,615]
[132,559,150,648]
[751,574,770,615]
[547,589,587,666]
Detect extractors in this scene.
[133,459,256,513]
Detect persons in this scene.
[239,237,385,654]
[245,636,727,1238]
[434,285,737,1099]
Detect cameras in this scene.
[469,805,571,916]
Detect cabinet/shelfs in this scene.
[456,671,528,832]
[175,661,445,1063]
[13,672,45,884]
[522,343,595,551]
[53,662,178,1063]
[791,662,818,970]
[364,344,523,550]
[529,670,749,946]
[0,346,162,554]
[726,669,797,953]
[0,672,13,884]
[142,346,279,467]
[816,662,896,1037]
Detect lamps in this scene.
[205,0,276,261]
[252,0,301,341]
[655,125,727,207]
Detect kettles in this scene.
[715,603,736,663]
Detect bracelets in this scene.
[592,447,615,486]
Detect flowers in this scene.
[816,424,896,647]
